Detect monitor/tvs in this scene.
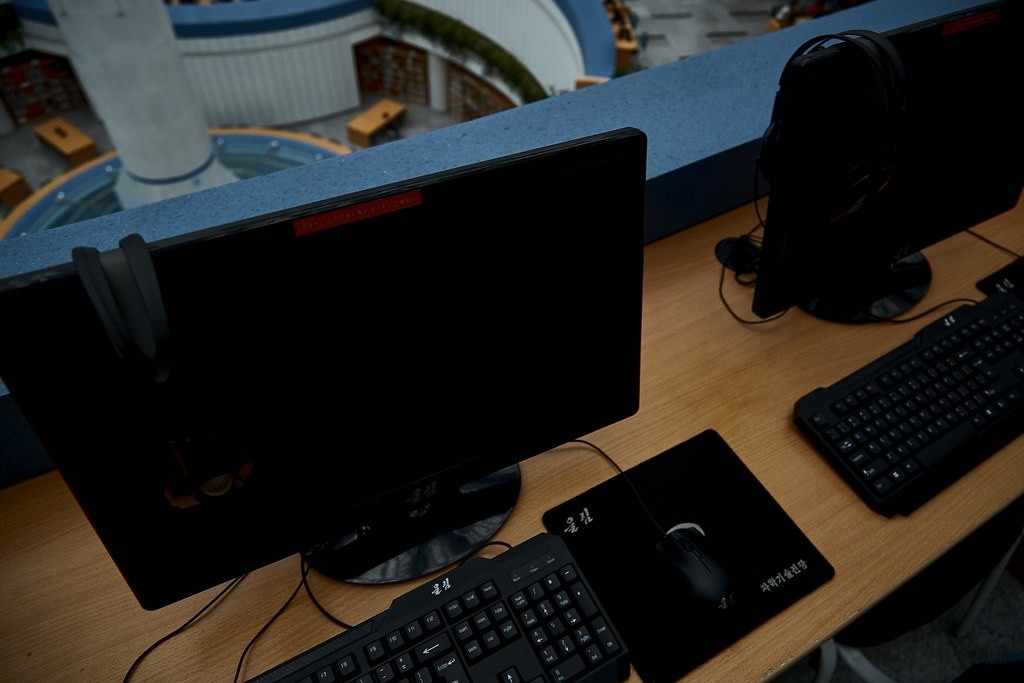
[0,126,647,612]
[754,0,1024,325]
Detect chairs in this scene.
[629,12,638,30]
[636,30,649,51]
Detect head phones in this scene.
[757,29,907,225]
[73,234,239,498]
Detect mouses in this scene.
[661,527,733,602]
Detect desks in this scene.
[598,0,640,72]
[345,96,406,149]
[0,185,1024,683]
[34,119,100,169]
[0,168,26,212]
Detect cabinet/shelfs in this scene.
[0,48,87,131]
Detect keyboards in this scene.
[243,532,631,683]
[793,291,1024,518]
